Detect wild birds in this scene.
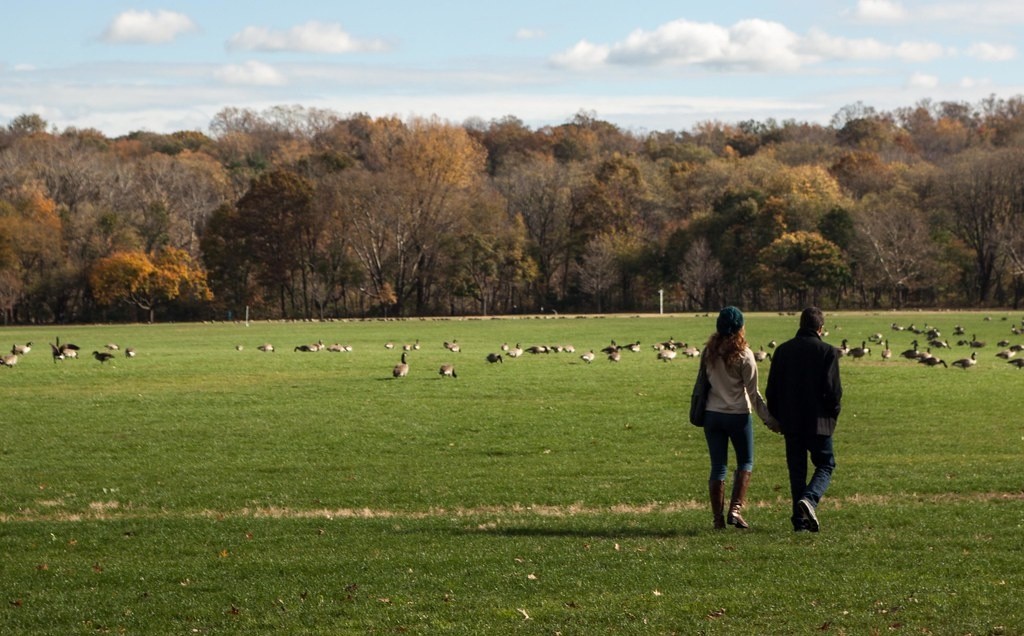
[817,314,1024,373]
[485,338,703,365]
[753,339,778,363]
[233,338,462,380]
[0,336,137,369]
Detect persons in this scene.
[688,306,780,529]
[764,307,843,532]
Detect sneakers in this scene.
[798,499,819,533]
[793,522,807,532]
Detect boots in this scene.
[727,470,752,528]
[706,479,726,530]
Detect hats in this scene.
[716,305,744,334]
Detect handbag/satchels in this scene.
[689,345,709,427]
